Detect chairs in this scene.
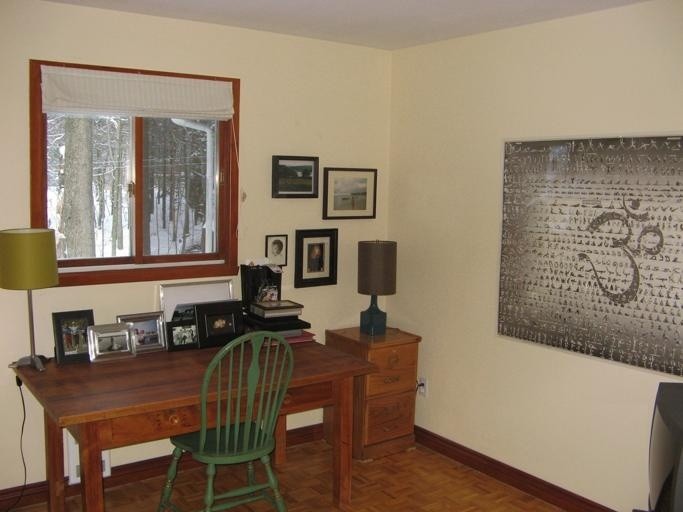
[158,332,294,512]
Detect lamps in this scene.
[358,241,396,335]
[0,227,57,373]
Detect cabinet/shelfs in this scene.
[326,325,421,461]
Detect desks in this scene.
[13,338,373,512]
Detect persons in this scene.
[68,323,80,352]
[134,329,144,346]
[63,322,73,352]
[177,329,193,344]
[208,317,233,336]
[268,240,285,264]
[107,337,122,352]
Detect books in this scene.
[244,331,316,347]
[277,329,302,337]
[250,300,304,318]
[245,319,311,330]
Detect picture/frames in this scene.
[161,280,236,323]
[272,155,319,199]
[265,234,287,267]
[197,300,247,347]
[52,309,94,365]
[87,323,137,362]
[164,319,199,351]
[294,230,338,289]
[117,310,168,355]
[323,168,377,219]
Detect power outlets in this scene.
[418,377,427,395]
